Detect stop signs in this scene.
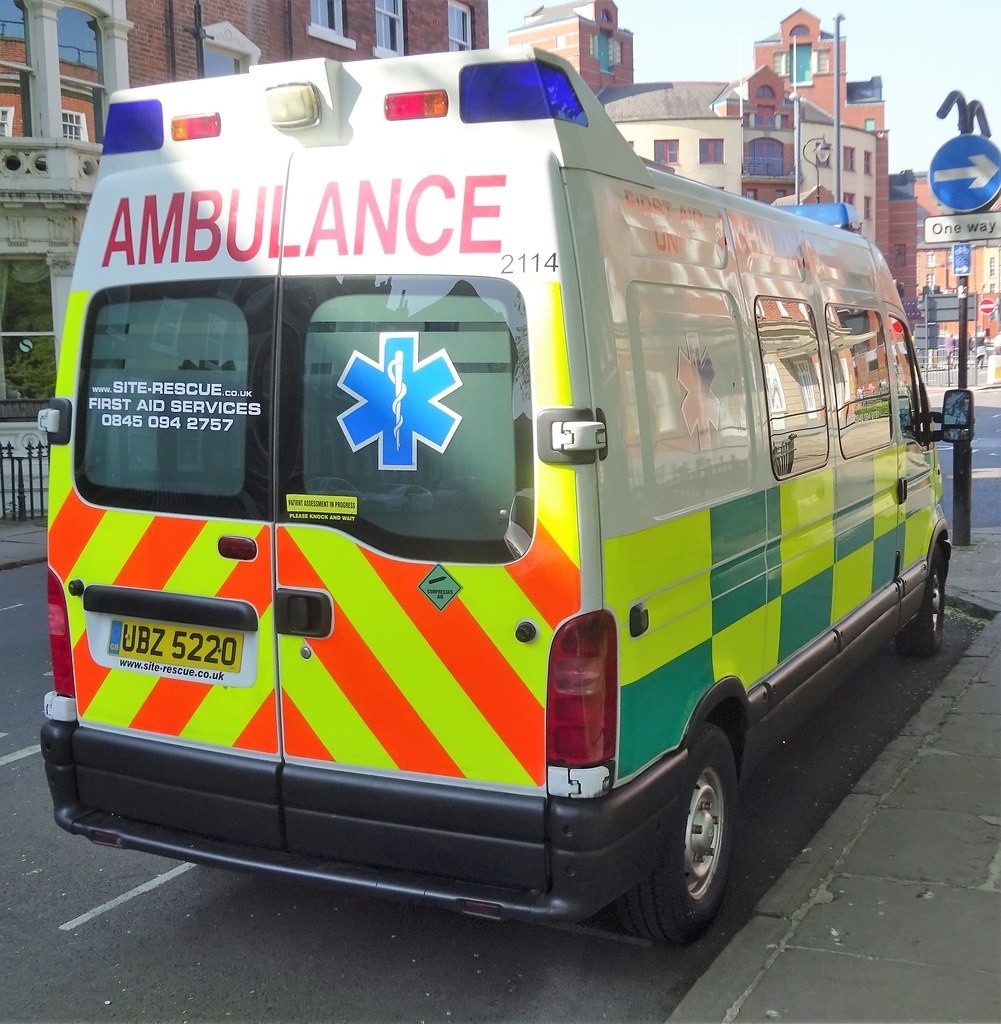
[979,298,996,315]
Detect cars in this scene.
[937,343,995,356]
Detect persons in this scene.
[994,332,1001,355]
[968,335,973,351]
[978,340,985,369]
[857,379,887,399]
[946,333,956,369]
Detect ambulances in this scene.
[36,40,974,946]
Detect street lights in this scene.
[802,131,832,203]
[831,12,847,202]
[788,92,804,208]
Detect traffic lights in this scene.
[897,280,904,298]
[934,285,940,293]
[923,286,929,296]
